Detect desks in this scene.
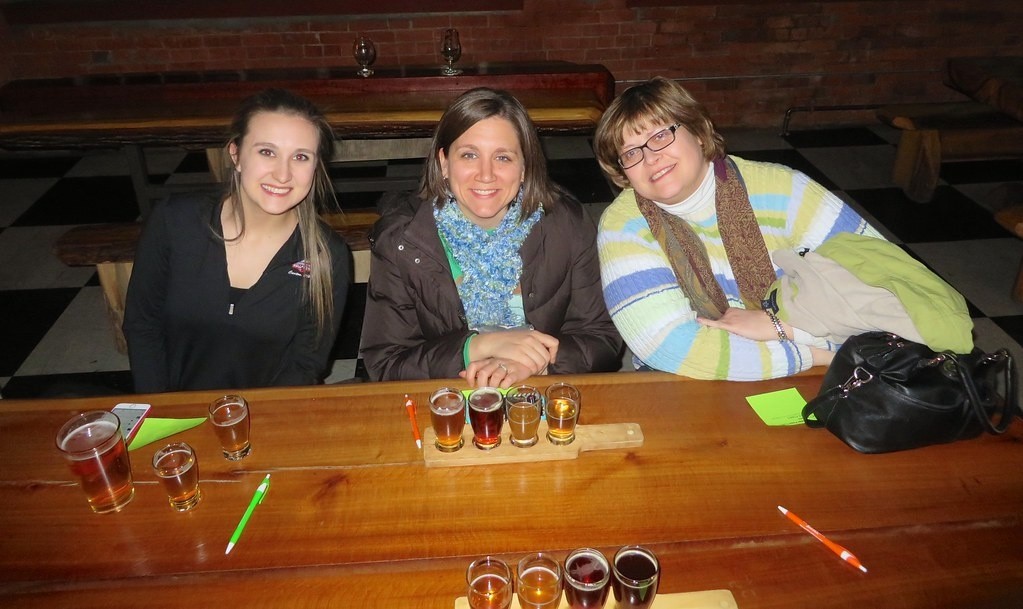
[1,73,623,269]
[0,348,1022,608]
[943,55,1023,126]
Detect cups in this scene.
[504,385,542,448]
[56,410,134,516]
[516,552,563,609]
[466,555,513,609]
[429,387,467,453]
[544,382,582,445]
[208,394,252,461]
[468,388,505,451]
[611,544,660,609]
[151,442,201,512]
[563,547,612,609]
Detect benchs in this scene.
[53,189,589,320]
[881,115,1022,303]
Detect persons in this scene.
[121,88,356,396]
[593,75,975,382]
[358,85,625,389]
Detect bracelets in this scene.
[764,309,788,340]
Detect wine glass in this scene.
[353,39,376,78]
[440,29,463,76]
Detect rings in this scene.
[498,363,509,374]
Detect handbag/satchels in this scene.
[97,260,137,355]
[801,329,1019,454]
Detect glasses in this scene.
[617,121,681,169]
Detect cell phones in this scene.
[111,402,152,446]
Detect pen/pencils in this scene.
[404,393,423,451]
[226,474,273,557]
[776,503,868,575]
